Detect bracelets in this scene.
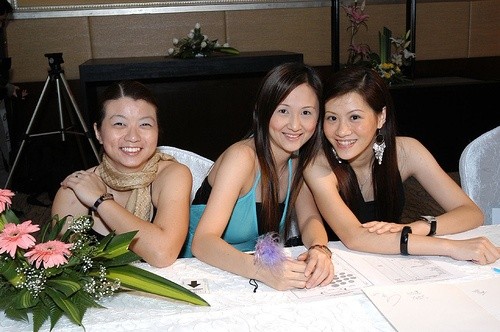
[308,245,332,259]
[400,226,412,255]
[93,193,114,214]
[419,215,437,236]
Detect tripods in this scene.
[4,53,102,191]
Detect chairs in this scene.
[459,126,500,225]
[155,145,212,203]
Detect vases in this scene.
[193,48,207,57]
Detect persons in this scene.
[303,67,500,266]
[178,62,334,291]
[52,80,193,267]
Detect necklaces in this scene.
[358,173,370,191]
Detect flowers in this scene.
[166,25,236,58]
[340,0,415,84]
[0,189,215,332]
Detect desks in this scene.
[0,225,500,332]
[375,75,500,173]
[78,49,304,168]
